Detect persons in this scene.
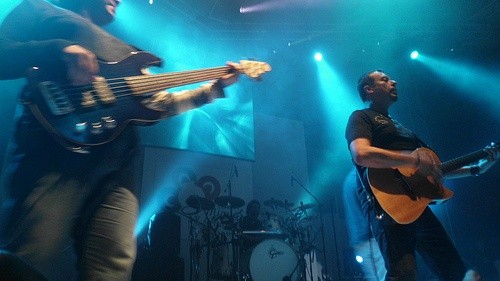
[232,200,265,281]
[0,0,241,281]
[345,70,500,281]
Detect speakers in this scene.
[133,211,184,281]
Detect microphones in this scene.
[234,165,239,178]
[290,175,294,185]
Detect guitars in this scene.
[26,51,272,149]
[363,142,500,225]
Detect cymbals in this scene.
[291,204,314,211]
[187,197,215,210]
[279,203,294,207]
[215,196,246,208]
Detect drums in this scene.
[242,231,286,254]
[264,213,283,231]
[240,239,300,281]
